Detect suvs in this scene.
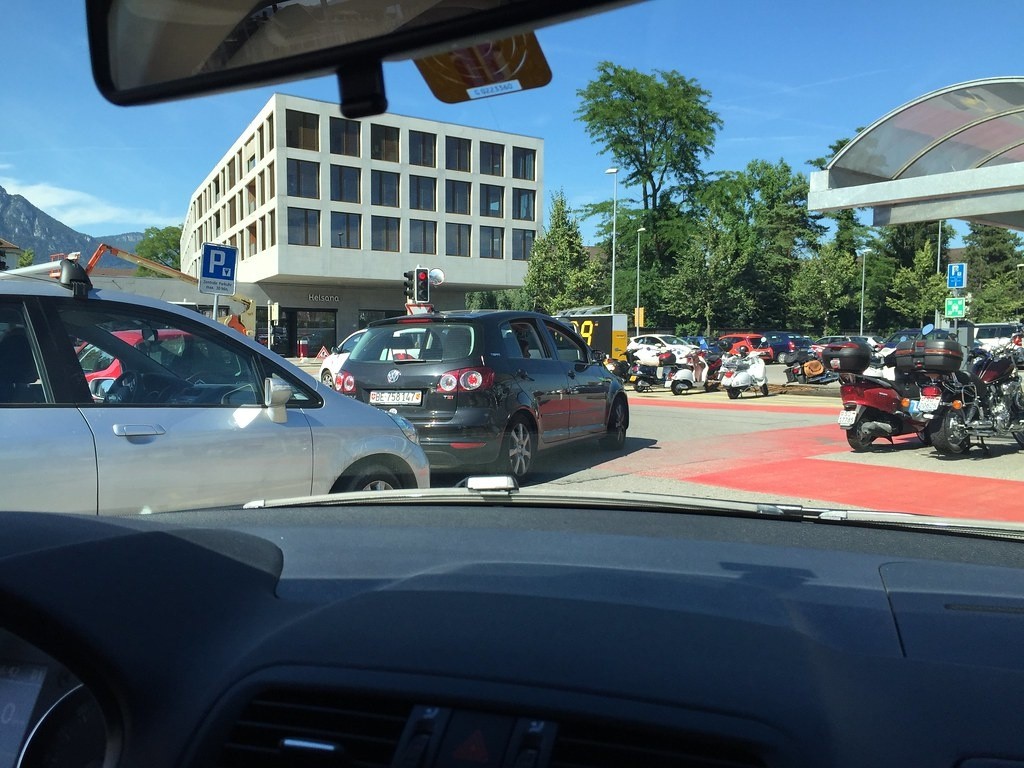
[973,322,1024,353]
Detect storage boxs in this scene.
[659,352,676,363]
[822,341,872,374]
[718,340,733,351]
[895,340,963,373]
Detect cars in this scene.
[75,328,196,388]
[626,328,949,368]
[318,329,369,391]
[334,309,629,478]
[2,279,431,519]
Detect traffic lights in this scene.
[415,268,429,303]
[403,271,415,304]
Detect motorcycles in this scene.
[822,324,934,452]
[895,335,1024,458]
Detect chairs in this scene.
[1,328,48,404]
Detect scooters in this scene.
[606,337,770,399]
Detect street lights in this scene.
[1015,263,1024,322]
[604,168,619,315]
[636,227,648,337]
[858,251,866,336]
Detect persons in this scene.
[518,339,532,358]
